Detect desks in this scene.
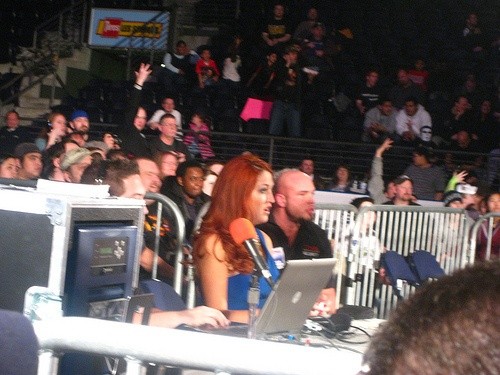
[301,318,386,354]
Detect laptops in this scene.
[175,258,337,337]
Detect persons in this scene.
[193,155,282,316]
[80,159,231,329]
[257,168,337,317]
[0,62,500,267]
[360,261,500,375]
[61,4,500,145]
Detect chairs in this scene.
[0,310,39,375]
[140,279,187,311]
[381,251,420,303]
[196,0,500,194]
[0,0,75,103]
[407,251,446,283]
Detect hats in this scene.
[311,21,326,28]
[283,46,298,55]
[14,143,43,158]
[59,148,92,171]
[70,110,88,121]
[394,174,414,185]
[84,141,108,154]
[200,163,224,197]
[442,190,465,207]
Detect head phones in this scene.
[297,312,350,334]
